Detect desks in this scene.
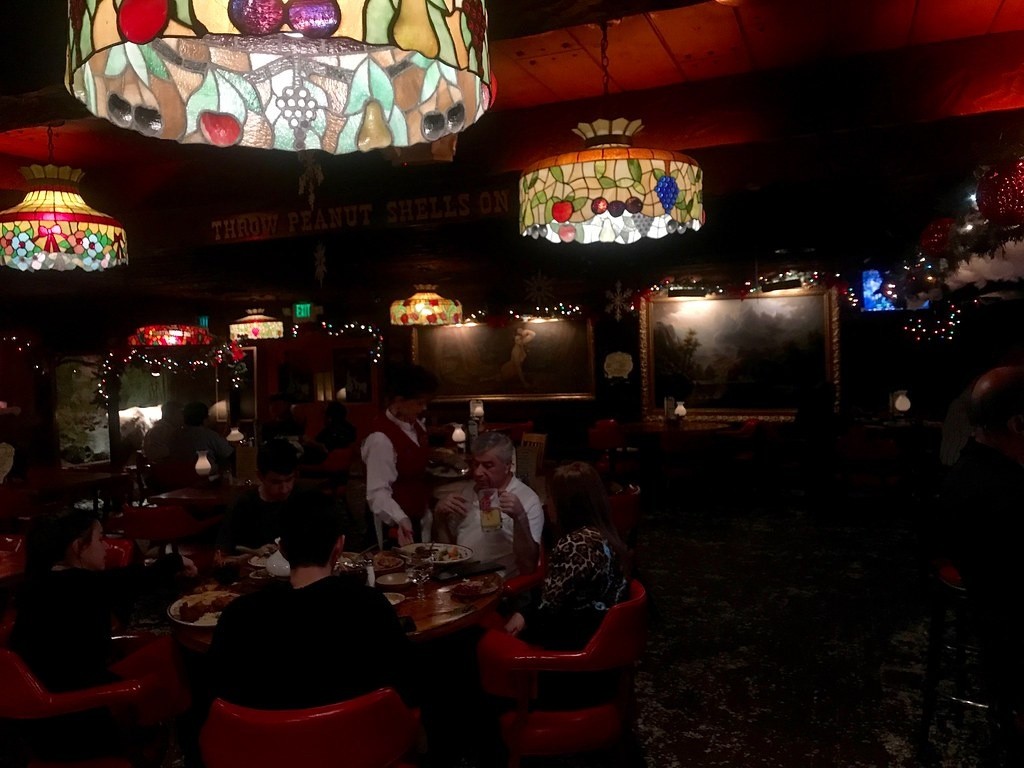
[42,468,133,528]
[426,421,535,449]
[620,420,727,521]
[864,420,944,494]
[148,487,238,509]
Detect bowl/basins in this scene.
[376,573,412,590]
[373,557,405,576]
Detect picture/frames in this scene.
[407,303,598,403]
[637,258,840,422]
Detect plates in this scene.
[399,543,474,564]
[247,555,270,569]
[167,591,240,626]
[451,581,499,597]
[383,592,404,605]
[249,570,274,579]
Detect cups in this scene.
[478,487,502,533]
[664,396,676,422]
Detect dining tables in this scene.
[173,554,500,656]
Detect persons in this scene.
[940,366,1024,675]
[11,365,634,764]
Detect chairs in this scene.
[0,649,167,768]
[519,432,557,523]
[200,686,422,768]
[478,578,649,768]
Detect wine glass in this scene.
[406,555,434,602]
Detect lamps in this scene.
[469,398,485,427]
[519,18,706,244]
[895,390,911,419]
[226,427,244,447]
[390,283,462,326]
[674,401,687,423]
[228,311,284,341]
[194,450,212,486]
[0,0,493,272]
